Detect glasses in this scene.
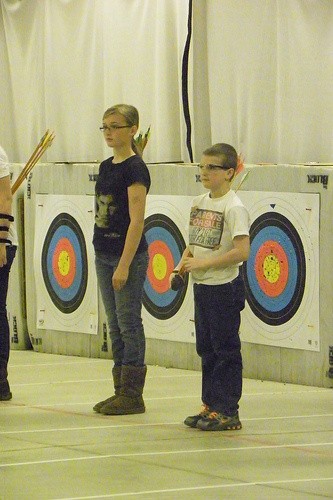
[99,124,132,132]
[199,164,230,172]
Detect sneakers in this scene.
[183,405,213,428]
[198,410,242,430]
[0,379,12,401]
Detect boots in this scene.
[93,367,121,412]
[100,365,147,414]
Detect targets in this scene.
[139,213,189,320]
[239,211,307,327]
[40,213,89,314]
